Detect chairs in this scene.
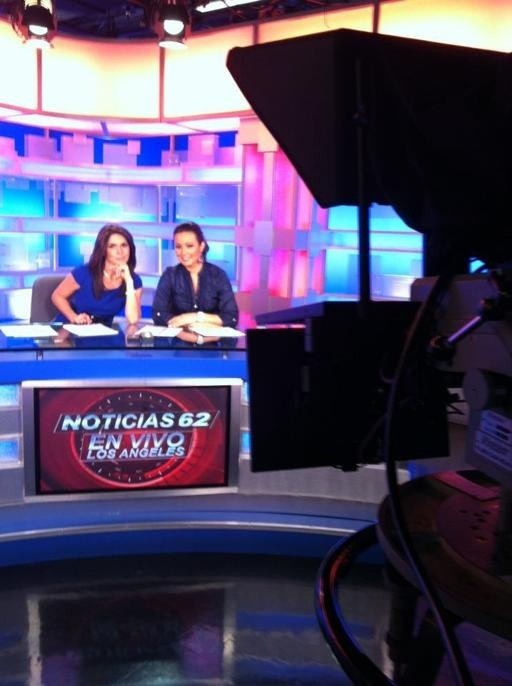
[28,275,71,323]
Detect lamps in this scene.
[6,1,59,53]
[152,0,194,51]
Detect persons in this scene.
[53,326,141,348]
[49,222,144,326]
[177,334,222,346]
[150,221,237,333]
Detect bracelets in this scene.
[197,311,205,321]
[197,335,203,345]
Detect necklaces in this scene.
[103,268,110,279]
